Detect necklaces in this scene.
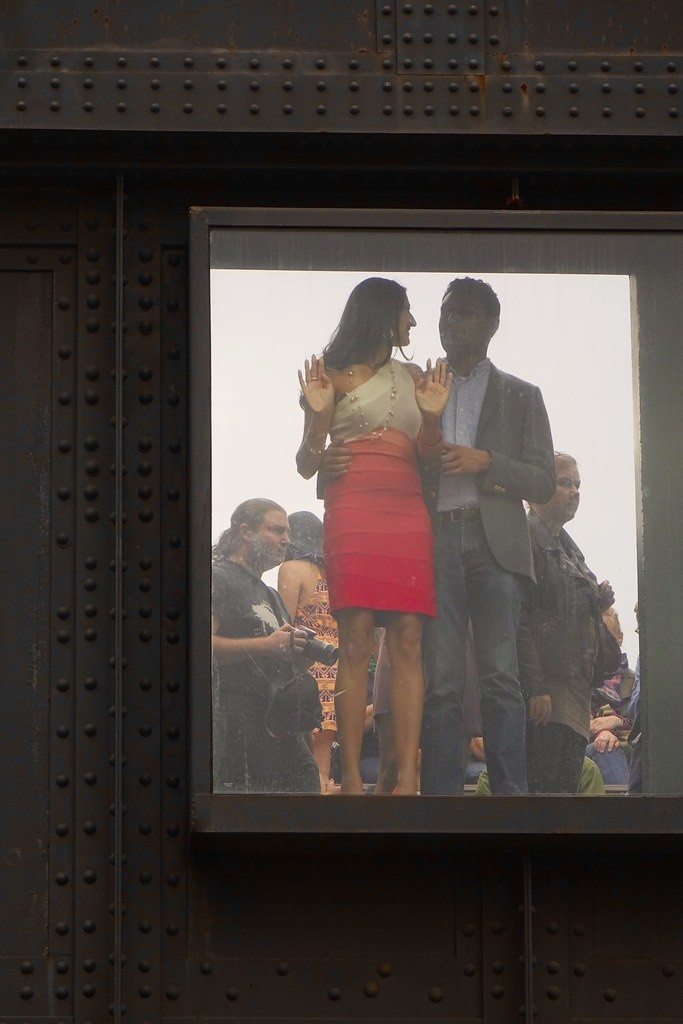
[346,355,397,437]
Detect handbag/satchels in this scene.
[276,672,323,735]
[590,608,622,687]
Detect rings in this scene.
[311,376,319,384]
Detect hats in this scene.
[288,511,323,557]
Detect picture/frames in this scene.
[187,208,683,834]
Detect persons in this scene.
[212,498,326,795]
[515,446,616,793]
[356,626,421,792]
[316,278,559,793]
[464,601,649,794]
[295,274,455,793]
[275,508,340,796]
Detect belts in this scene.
[442,508,480,522]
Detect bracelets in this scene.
[419,427,444,447]
[615,714,624,730]
[307,429,326,455]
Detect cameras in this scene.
[296,625,338,667]
